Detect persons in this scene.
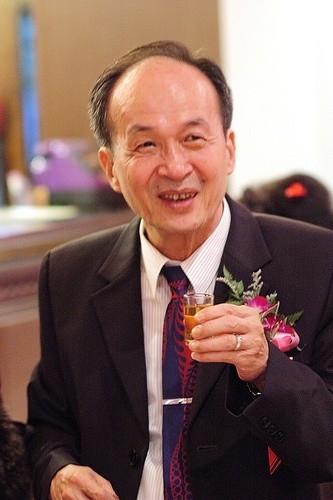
[25,39,333,500]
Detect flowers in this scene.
[215,265,303,357]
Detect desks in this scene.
[0,207,137,427]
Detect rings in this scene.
[233,333,242,350]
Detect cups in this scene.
[184,292,214,345]
